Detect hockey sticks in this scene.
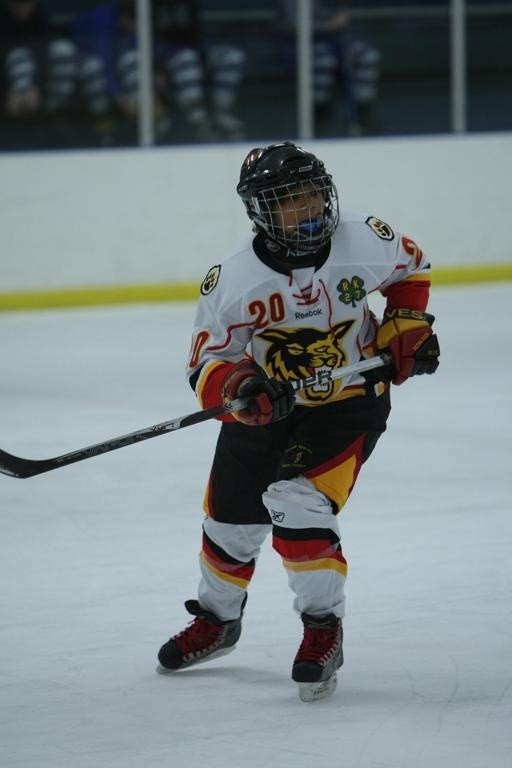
[0,353,397,478]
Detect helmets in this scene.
[236,140,333,254]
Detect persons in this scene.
[1,2,512,148]
[156,140,440,702]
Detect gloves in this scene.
[217,358,296,427]
[375,307,440,386]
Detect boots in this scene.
[291,610,345,683]
[154,590,249,670]
